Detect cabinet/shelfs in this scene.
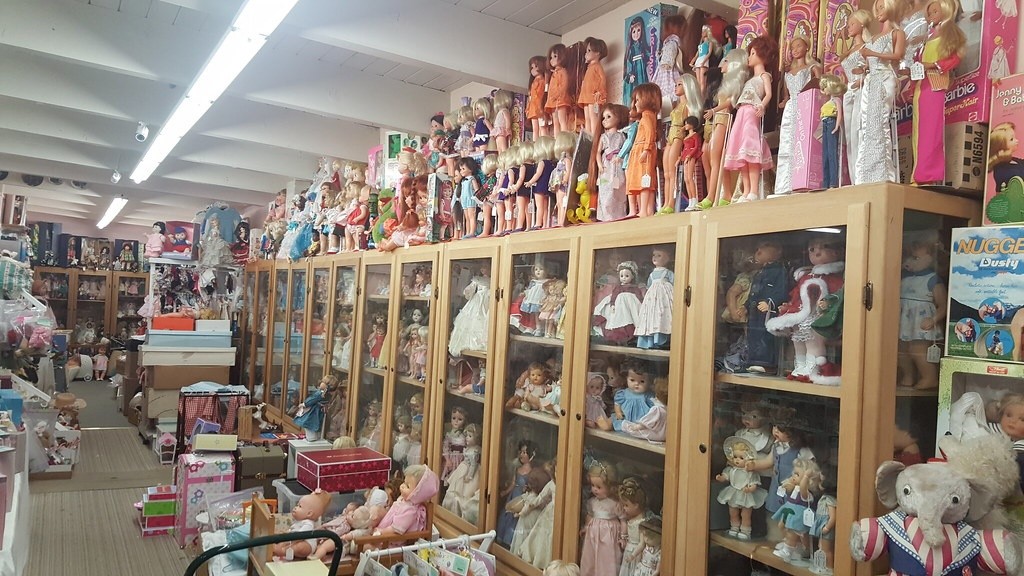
[238,257,273,417]
[301,250,362,443]
[484,211,692,576]
[675,181,980,576]
[266,256,311,436]
[111,271,149,338]
[427,235,504,544]
[350,244,443,486]
[33,266,113,352]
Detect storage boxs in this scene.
[137,344,237,367]
[935,357,1024,461]
[824,0,1021,137]
[235,446,286,499]
[509,92,532,148]
[123,379,141,417]
[898,121,989,194]
[148,486,176,500]
[622,4,680,108]
[563,40,588,133]
[147,364,231,390]
[297,444,391,492]
[77,236,99,267]
[145,330,232,348]
[161,221,200,260]
[145,517,174,528]
[943,226,1024,365]
[138,518,175,537]
[142,495,174,517]
[174,451,236,547]
[114,239,139,268]
[145,387,180,418]
[125,351,140,378]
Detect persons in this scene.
[393,261,433,466]
[201,213,250,266]
[117,278,146,338]
[714,229,947,390]
[982,393,1024,483]
[578,242,676,576]
[74,276,105,344]
[324,267,354,443]
[276,464,439,561]
[91,345,110,380]
[10,195,192,270]
[261,0,1023,259]
[356,266,390,452]
[33,273,68,330]
[293,375,339,442]
[440,259,491,528]
[495,256,569,571]
[715,394,922,575]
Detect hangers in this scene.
[374,532,473,571]
[183,391,213,396]
[231,390,247,398]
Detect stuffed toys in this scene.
[846,430,1023,576]
